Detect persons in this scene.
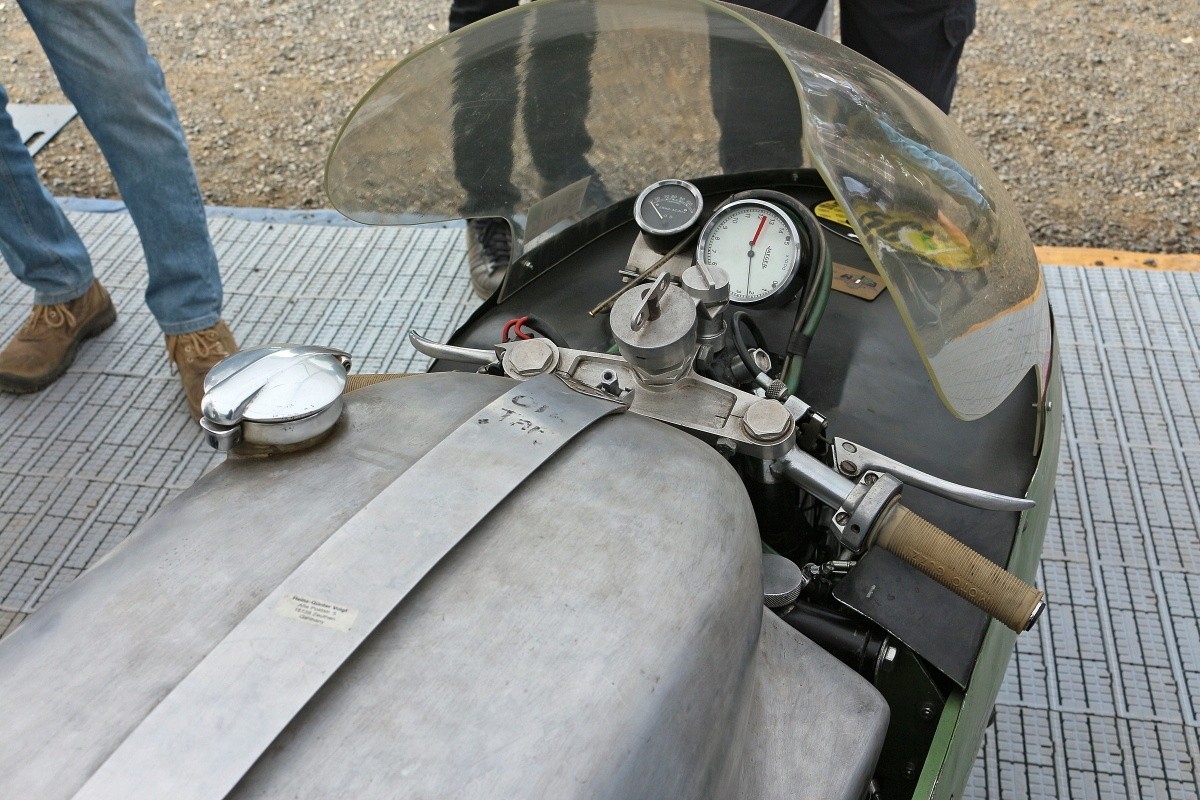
[708,0,975,173]
[448,0,613,299]
[1,0,239,420]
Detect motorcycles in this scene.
[1,0,1071,800]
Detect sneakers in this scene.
[468,213,524,300]
[163,318,238,422]
[538,169,611,223]
[0,278,116,396]
